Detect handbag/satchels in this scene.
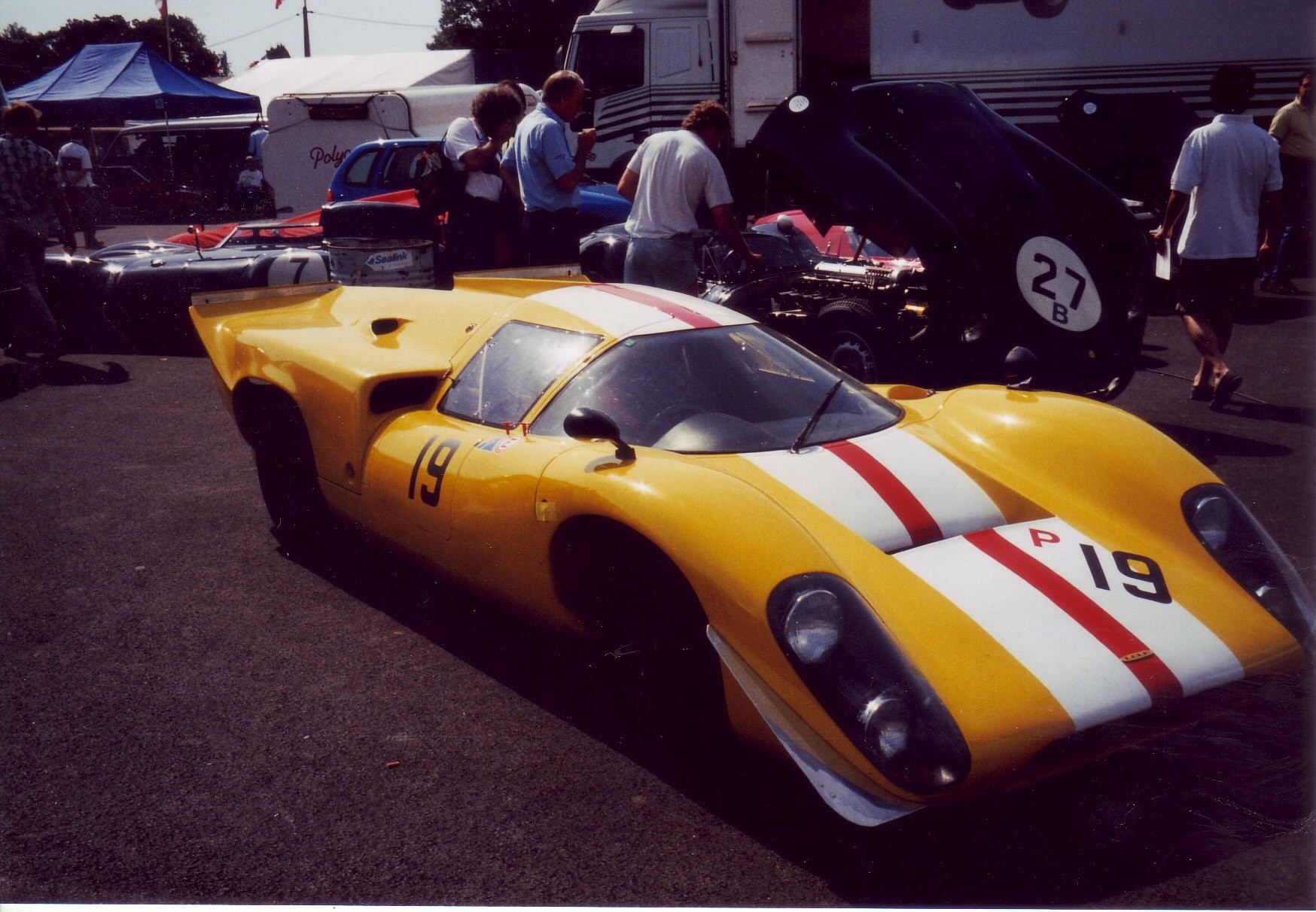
[413,116,481,216]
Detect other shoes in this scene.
[85,240,104,248]
[4,346,28,361]
[65,242,76,249]
[1260,278,1298,295]
[41,335,67,356]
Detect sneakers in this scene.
[1189,386,1214,401]
[1209,371,1243,410]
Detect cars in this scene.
[328,137,634,269]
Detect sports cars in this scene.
[34,223,329,344]
[190,274,1314,837]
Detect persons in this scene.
[446,88,523,272]
[57,124,105,250]
[1251,67,1316,296]
[236,155,264,220]
[497,70,595,267]
[1144,62,1287,408]
[0,99,72,367]
[616,99,766,306]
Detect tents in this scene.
[203,49,479,112]
[0,33,264,217]
